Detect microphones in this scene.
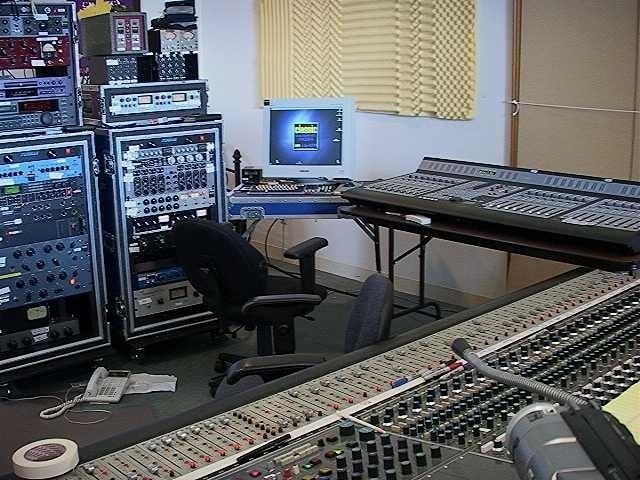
[449,336,589,408]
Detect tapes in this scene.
[11,438,79,479]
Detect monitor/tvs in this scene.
[262,98,353,186]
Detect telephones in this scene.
[83,367,131,402]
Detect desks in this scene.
[337,206,637,331]
